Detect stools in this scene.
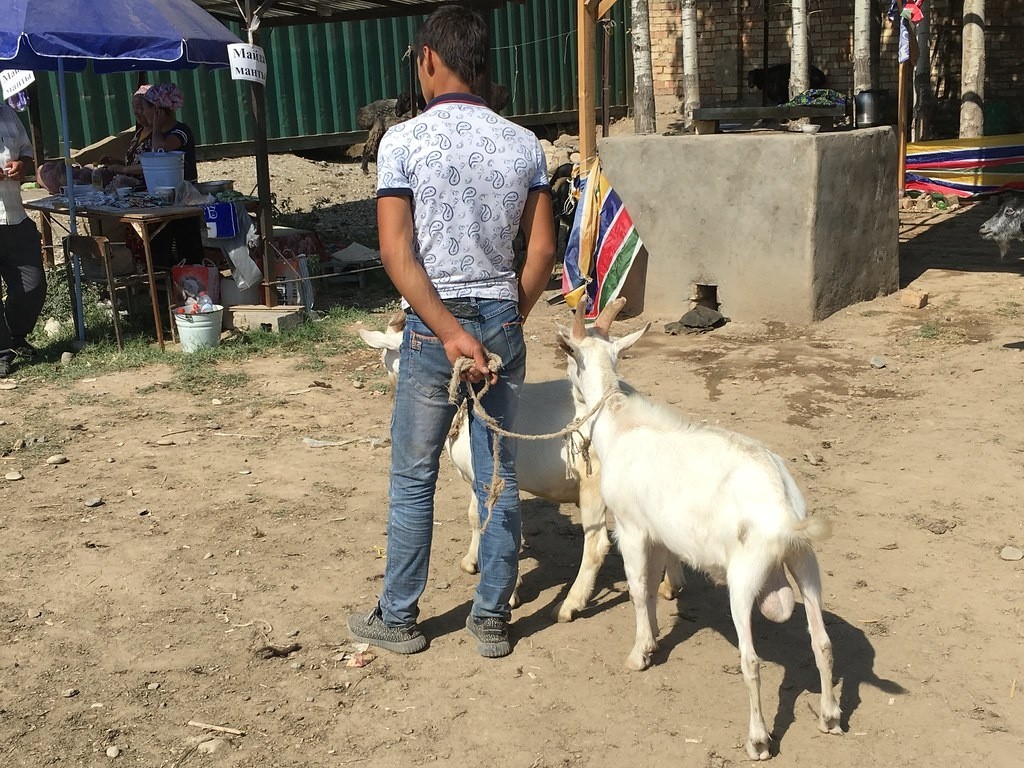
[319,253,382,290]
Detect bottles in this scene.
[938,200,945,209]
[177,292,214,312]
[91,161,103,193]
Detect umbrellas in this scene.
[0,0,248,340]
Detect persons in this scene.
[0,103,48,379]
[346,6,556,659]
[97,82,203,268]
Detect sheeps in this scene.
[360,317,685,622]
[979,193,1024,260]
[555,294,845,763]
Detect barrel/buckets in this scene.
[172,303,224,352]
[140,148,186,202]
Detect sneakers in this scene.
[466,614,510,657]
[347,601,427,653]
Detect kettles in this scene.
[853,89,887,128]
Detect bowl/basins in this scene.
[802,124,820,135]
[116,187,131,196]
[156,187,176,206]
[196,180,235,195]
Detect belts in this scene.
[404,298,490,319]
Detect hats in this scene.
[133,84,154,98]
[143,83,184,111]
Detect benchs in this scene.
[692,104,845,134]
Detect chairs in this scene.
[62,234,180,354]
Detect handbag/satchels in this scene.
[256,249,314,311]
[171,258,219,306]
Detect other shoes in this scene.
[11,337,38,357]
[0,360,11,377]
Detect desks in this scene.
[200,224,330,271]
[21,194,259,343]
